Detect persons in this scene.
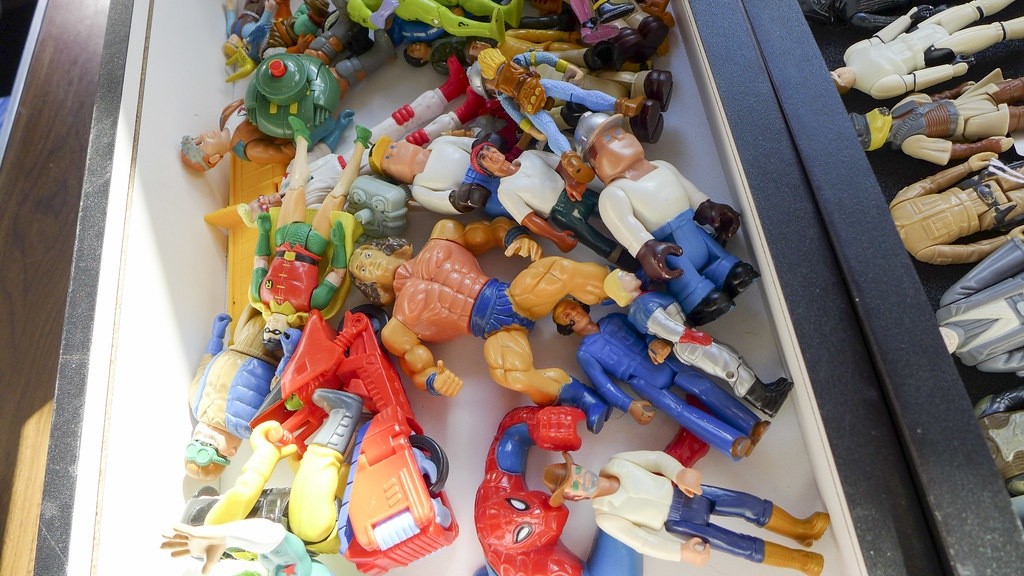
[828,0,1024,531]
[159,0,830,576]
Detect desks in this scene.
[32,1,949,576]
